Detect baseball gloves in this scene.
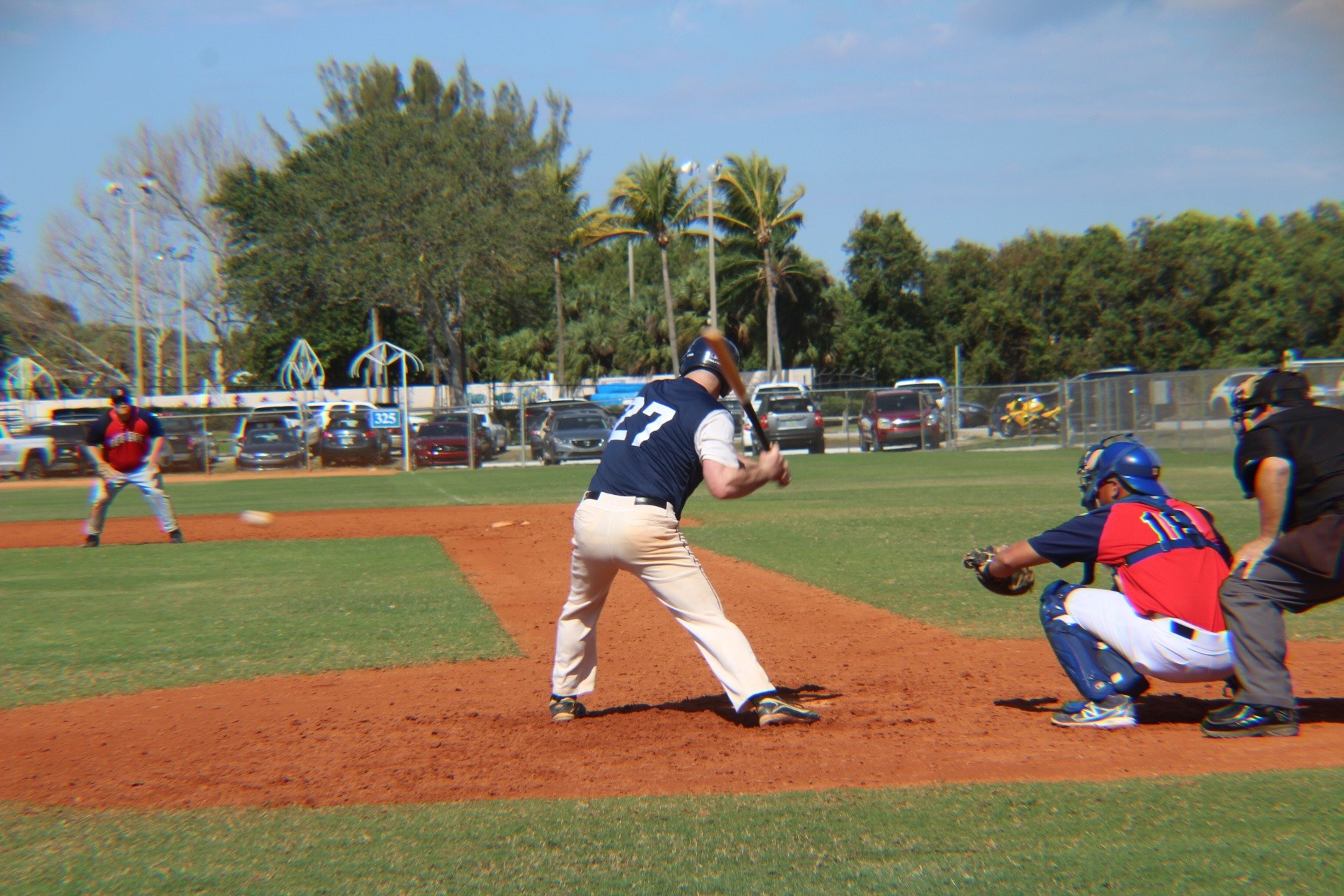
[96,461,124,491]
[957,545,1038,595]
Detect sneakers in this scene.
[549,696,585,721]
[754,696,820,726]
[1051,695,1137,728]
[1201,702,1300,737]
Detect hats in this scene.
[111,388,130,403]
[1257,370,1316,407]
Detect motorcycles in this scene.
[997,395,1067,439]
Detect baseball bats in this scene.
[704,327,795,488]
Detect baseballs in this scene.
[242,507,276,531]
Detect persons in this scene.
[967,433,1238,729]
[1199,367,1344,737]
[548,334,823,727]
[82,386,185,546]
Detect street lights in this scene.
[162,243,194,396]
[107,179,150,397]
[681,158,724,334]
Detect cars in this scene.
[243,400,616,461]
[989,365,1178,433]
[541,408,618,466]
[237,414,307,471]
[1208,370,1344,420]
[319,411,392,467]
[152,412,220,474]
[410,420,485,470]
[0,405,114,481]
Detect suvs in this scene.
[715,381,814,452]
[895,376,956,442]
[858,386,942,453]
[750,393,825,457]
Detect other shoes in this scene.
[171,530,184,542]
[1061,700,1090,714]
[82,534,101,546]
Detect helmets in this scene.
[1101,442,1169,511]
[679,336,740,398]
